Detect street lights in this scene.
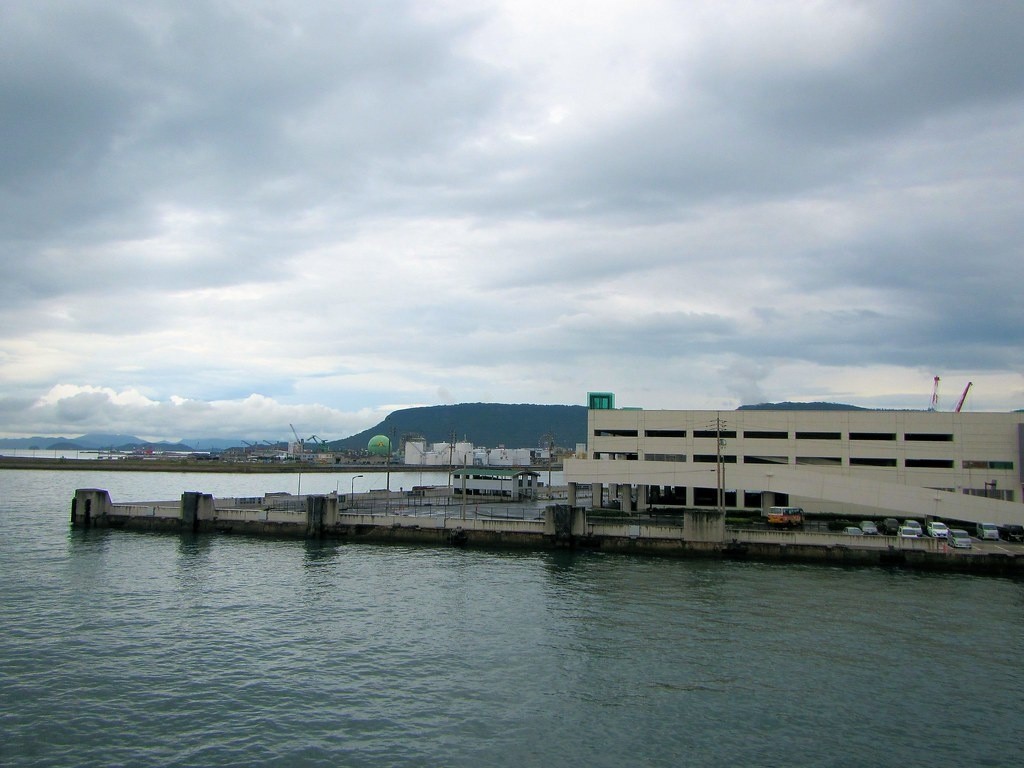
[352,475,363,509]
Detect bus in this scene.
[768,506,805,527]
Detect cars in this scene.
[897,520,922,538]
[927,522,949,540]
[828,518,900,535]
[947,529,972,549]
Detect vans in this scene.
[1003,524,1024,542]
[976,523,1000,541]
